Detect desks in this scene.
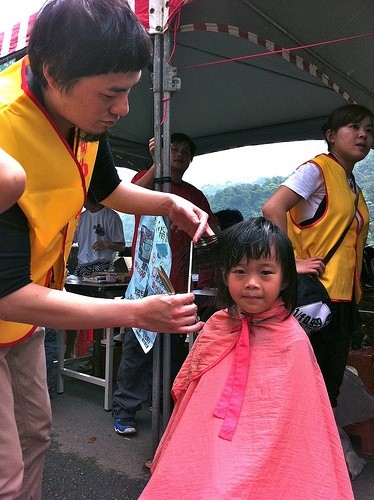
[57,275,128,411]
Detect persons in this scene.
[0,0,217,500]
[110,133,222,437]
[262,104,373,410]
[62,190,126,371]
[134,216,357,500]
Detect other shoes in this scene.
[114,418,137,435]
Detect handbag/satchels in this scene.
[291,273,335,335]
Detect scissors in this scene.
[155,266,199,335]
